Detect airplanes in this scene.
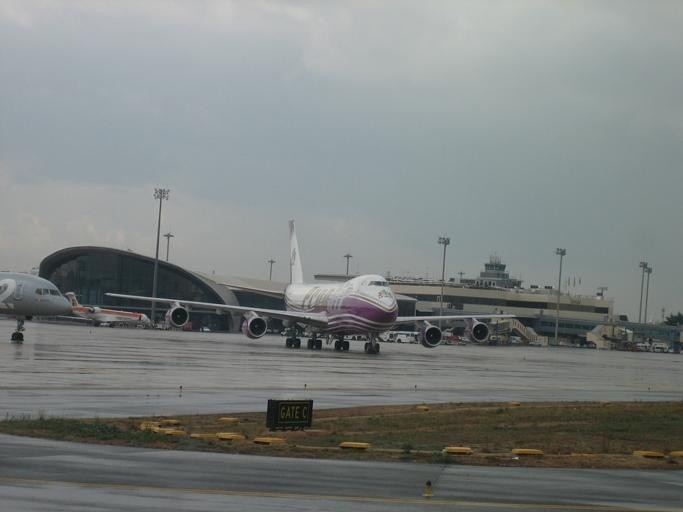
[397,313,538,348]
[61,289,150,330]
[0,269,72,343]
[104,216,520,354]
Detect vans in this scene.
[279,327,298,337]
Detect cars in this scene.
[200,326,211,332]
[100,320,113,327]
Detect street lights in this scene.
[553,246,565,346]
[643,267,653,324]
[342,253,353,276]
[148,185,171,329]
[437,236,451,331]
[637,261,646,326]
[267,259,277,280]
[163,232,174,262]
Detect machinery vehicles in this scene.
[181,321,194,333]
